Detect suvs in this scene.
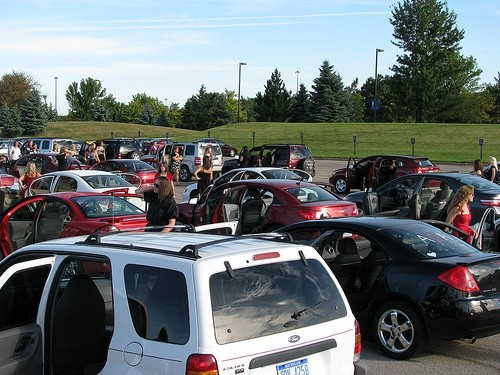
[0,223,361,375]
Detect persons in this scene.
[10,140,251,234]
[444,185,477,246]
[488,155,498,183]
[474,159,483,177]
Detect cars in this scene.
[481,161,500,186]
[181,166,326,205]
[174,177,362,241]
[257,215,500,360]
[0,134,239,211]
[26,169,150,214]
[0,190,149,261]
[342,171,500,256]
[328,154,445,196]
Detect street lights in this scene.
[237,62,248,123]
[53,76,58,116]
[373,48,384,123]
[295,70,300,96]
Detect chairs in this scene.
[243,188,264,226]
[424,182,450,219]
[37,205,63,240]
[52,274,106,375]
[363,241,387,290]
[397,189,433,217]
[262,152,272,167]
[331,237,364,299]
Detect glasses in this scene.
[154,183,159,188]
